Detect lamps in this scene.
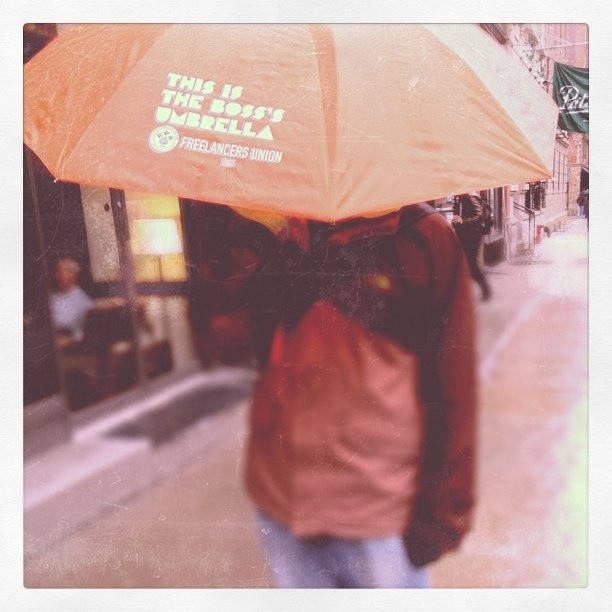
[131,218,184,338]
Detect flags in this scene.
[549,59,589,133]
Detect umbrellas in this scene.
[23,24,565,225]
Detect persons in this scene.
[47,253,95,360]
[449,189,494,304]
[576,193,586,218]
[201,188,479,589]
